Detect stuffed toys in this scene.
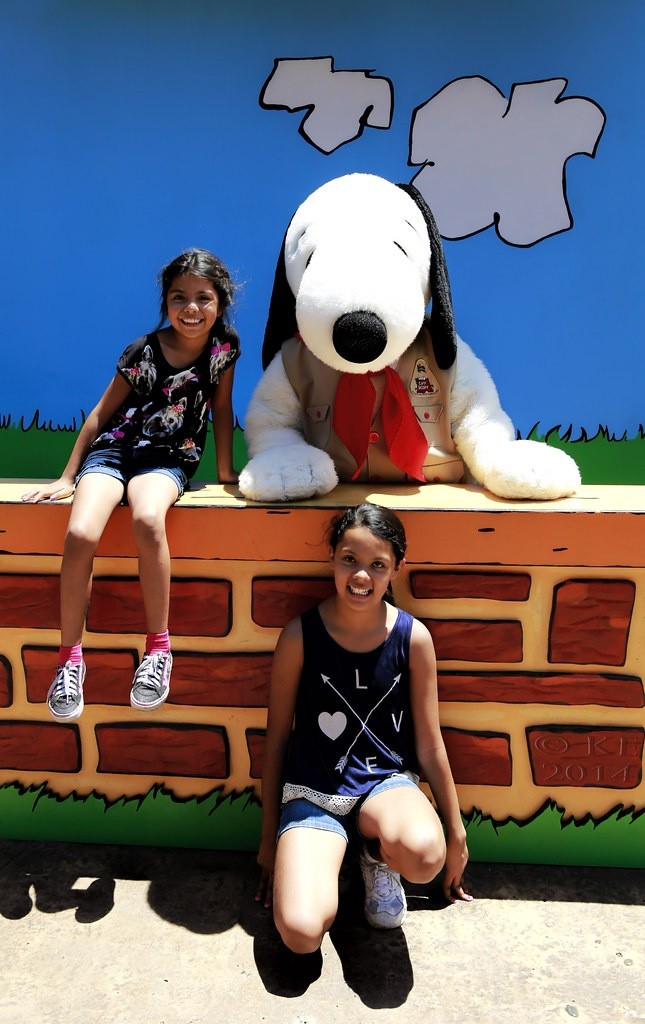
[234,162,588,509]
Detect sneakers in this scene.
[359,854,408,929]
[47,659,87,724]
[130,651,172,711]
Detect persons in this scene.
[23,231,245,720]
[248,500,475,961]
